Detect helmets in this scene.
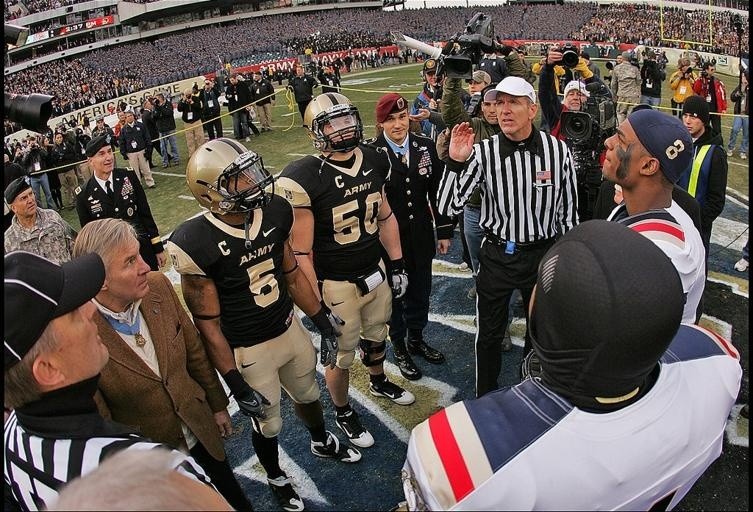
[186,138,274,216]
[304,93,363,152]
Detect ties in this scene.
[105,181,117,206]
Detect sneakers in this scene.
[727,147,735,157]
[335,405,375,448]
[311,430,362,462]
[501,329,511,351]
[734,258,749,271]
[739,152,747,159]
[268,471,305,511]
[458,262,471,271]
[468,285,476,300]
[370,374,415,405]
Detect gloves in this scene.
[309,308,338,370]
[392,257,409,298]
[223,370,270,419]
[575,58,593,80]
[319,299,344,338]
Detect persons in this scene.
[2,0,753,512]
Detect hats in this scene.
[86,133,114,156]
[529,220,685,398]
[627,109,694,183]
[484,76,537,102]
[5,176,32,203]
[465,70,491,84]
[564,81,589,98]
[682,95,710,124]
[2,251,105,372]
[376,94,407,123]
[423,59,438,73]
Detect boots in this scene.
[391,336,422,379]
[408,327,444,364]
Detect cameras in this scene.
[3,92,56,135]
[604,75,612,81]
[554,44,580,69]
[31,143,35,148]
[700,71,708,76]
[185,93,191,99]
[204,81,210,85]
